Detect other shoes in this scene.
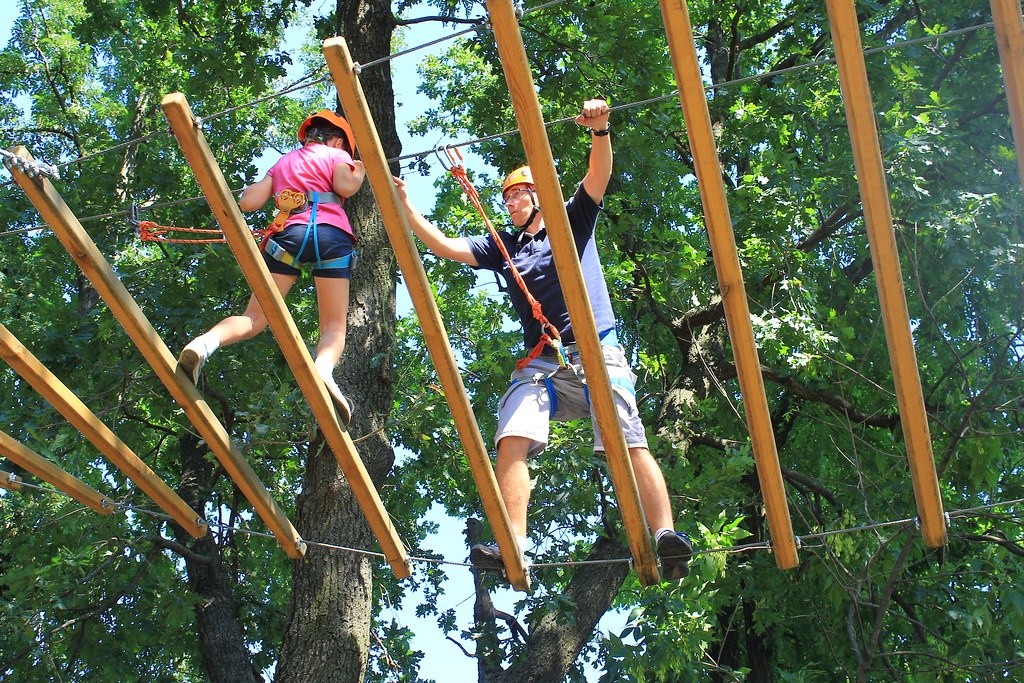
[657,532,693,580]
[177,340,205,387]
[314,368,351,428]
[470,545,512,585]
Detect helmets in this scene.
[502,166,535,198]
[297,110,355,161]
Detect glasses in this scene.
[501,188,536,209]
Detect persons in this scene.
[392,100,692,582]
[178,112,365,427]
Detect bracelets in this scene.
[593,123,612,135]
